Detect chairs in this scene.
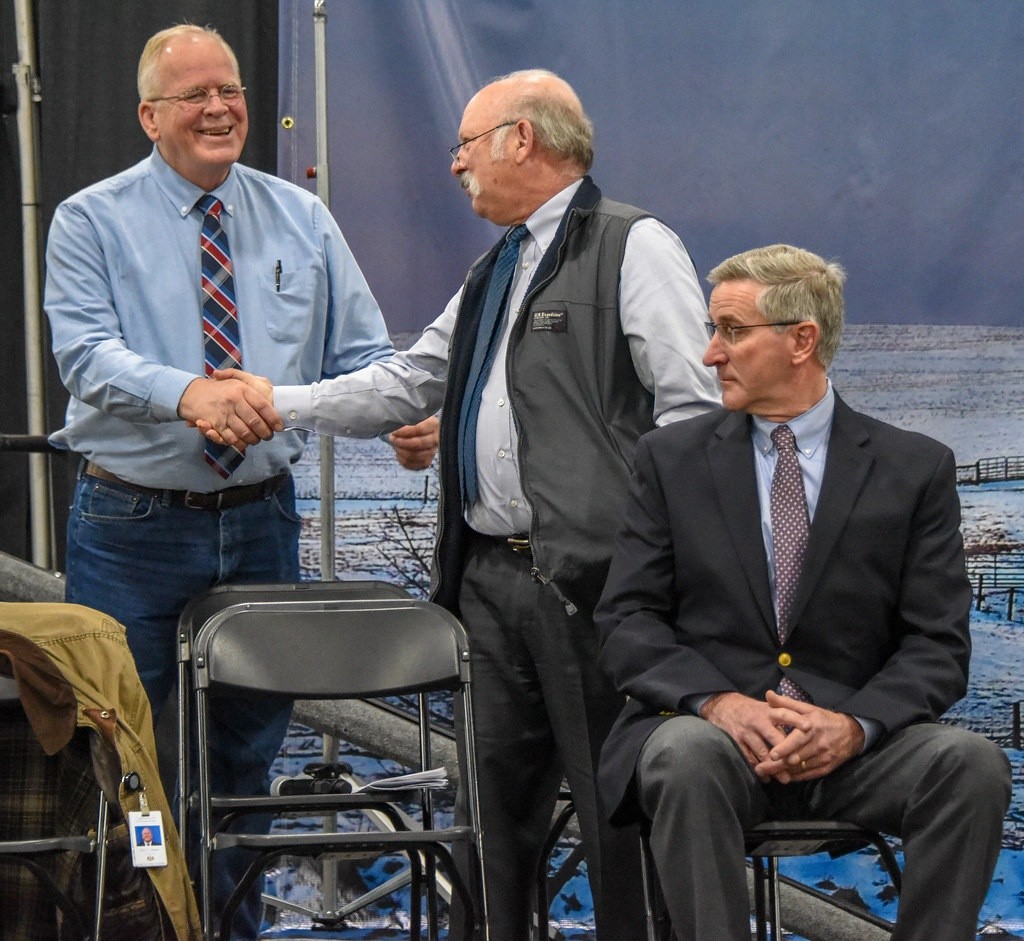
[1,565,915,940]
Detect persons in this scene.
[184,67,723,940]
[596,240,1012,941]
[137,827,160,845]
[44,21,439,940]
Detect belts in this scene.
[87,460,284,510]
[468,526,530,551]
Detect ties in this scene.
[194,194,247,481]
[457,224,530,517]
[770,426,812,734]
[147,843,150,846]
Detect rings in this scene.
[801,761,806,770]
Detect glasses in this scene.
[146,84,247,109]
[704,319,801,347]
[448,120,519,166]
